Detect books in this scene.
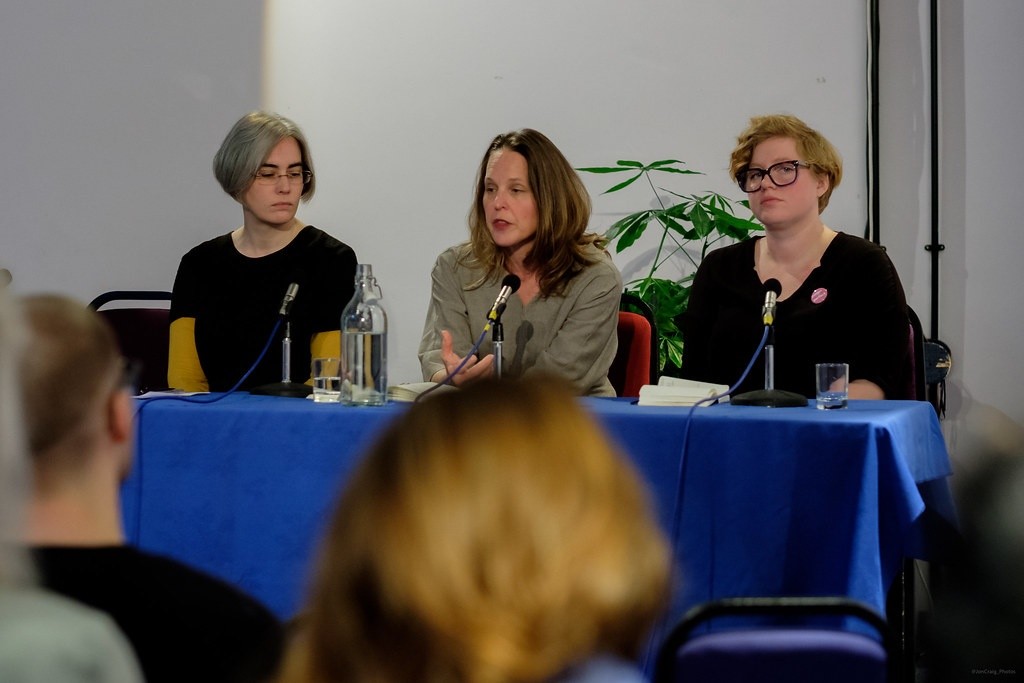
[638,376,730,406]
[387,381,458,402]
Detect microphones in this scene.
[484,274,521,331]
[278,267,307,318]
[761,277,782,326]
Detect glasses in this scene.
[736,159,811,193]
[252,169,314,186]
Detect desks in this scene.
[118,388,951,683]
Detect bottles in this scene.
[340,262,388,405]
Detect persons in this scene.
[0,291,283,683]
[281,376,671,683]
[417,128,623,398]
[681,115,910,401]
[166,113,359,394]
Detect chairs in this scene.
[607,293,661,398]
[645,595,910,683]
[85,291,173,396]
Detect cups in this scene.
[815,362,849,411]
[310,357,339,404]
